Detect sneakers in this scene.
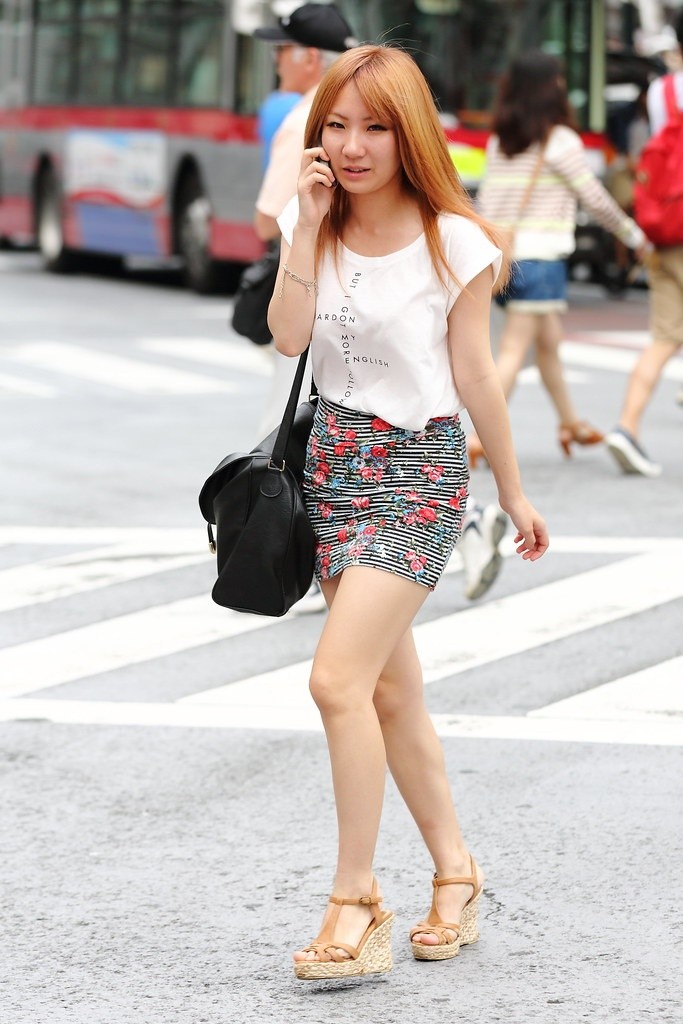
[454,497,508,600]
[607,428,663,477]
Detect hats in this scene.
[255,4,353,52]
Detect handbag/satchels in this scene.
[199,398,319,617]
[232,257,279,345]
[493,230,514,295]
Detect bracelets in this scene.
[279,266,318,298]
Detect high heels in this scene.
[560,421,604,454]
[468,432,490,468]
[409,853,483,961]
[293,875,395,980]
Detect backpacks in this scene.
[632,74,683,247]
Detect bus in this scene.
[1,0,681,295]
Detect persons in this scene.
[609,5,683,476]
[268,44,548,980]
[467,55,662,468]
[254,5,360,295]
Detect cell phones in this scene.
[318,144,329,185]
